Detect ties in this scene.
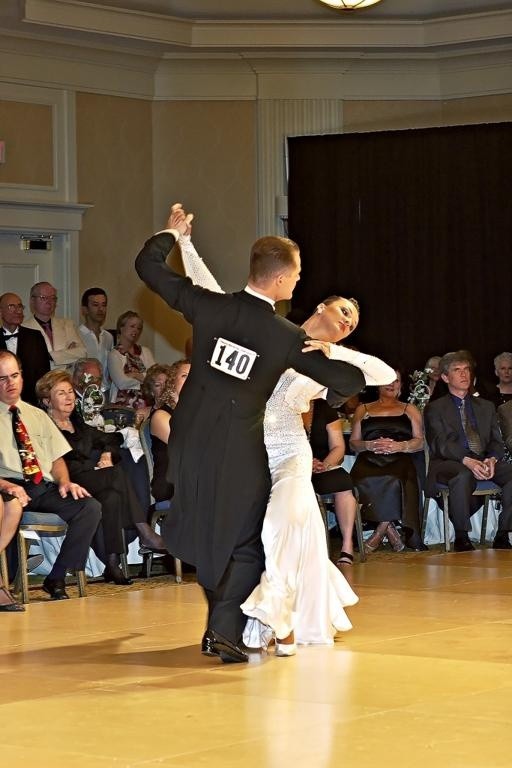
[459,399,482,455]
[9,405,44,486]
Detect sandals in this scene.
[386,527,404,551]
[337,551,354,567]
[363,531,384,553]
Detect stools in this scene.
[0,507,88,604]
[311,473,512,562]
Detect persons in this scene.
[0,281,201,613]
[168,203,398,656]
[135,211,364,664]
[300,346,511,567]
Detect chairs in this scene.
[136,416,183,582]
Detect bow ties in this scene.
[4,333,19,340]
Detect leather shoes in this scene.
[453,538,475,551]
[201,630,248,663]
[274,628,298,657]
[140,541,169,555]
[41,584,68,599]
[491,538,512,549]
[0,586,25,612]
[27,554,44,572]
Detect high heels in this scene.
[103,568,134,585]
